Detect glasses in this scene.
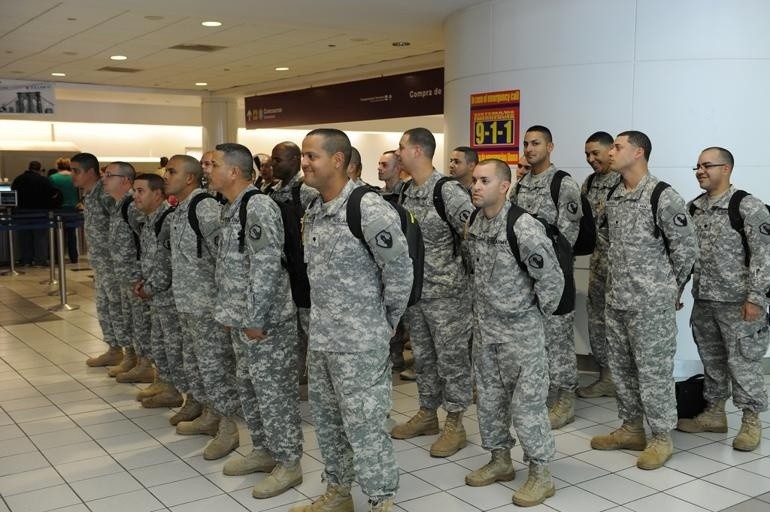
[103,171,127,180]
[692,163,726,173]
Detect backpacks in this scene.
[239,189,315,309]
[506,200,577,317]
[347,186,426,308]
[552,170,597,256]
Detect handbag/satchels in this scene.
[675,373,711,418]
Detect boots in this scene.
[638,435,676,470]
[575,367,619,399]
[170,394,239,461]
[87,351,184,407]
[733,409,764,451]
[430,412,467,458]
[512,465,556,507]
[679,398,730,434]
[464,449,516,487]
[224,447,393,512]
[390,406,439,440]
[591,415,648,452]
[547,390,576,429]
